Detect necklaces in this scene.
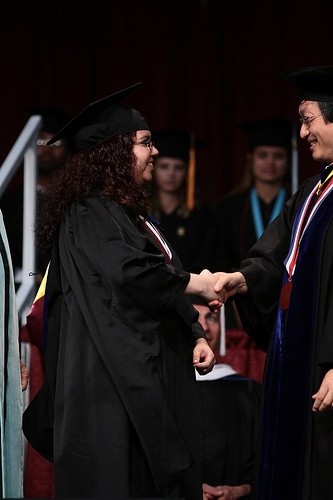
[284,173,333,284]
[140,214,175,263]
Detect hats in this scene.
[25,104,73,134]
[152,128,205,209]
[46,82,151,155]
[281,66,333,102]
[233,117,302,195]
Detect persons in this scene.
[34,81,228,500]
[209,66,333,500]
[183,262,264,500]
[146,126,213,259]
[0,105,76,261]
[216,115,301,311]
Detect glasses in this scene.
[36,138,63,147]
[132,137,155,150]
[298,107,332,125]
